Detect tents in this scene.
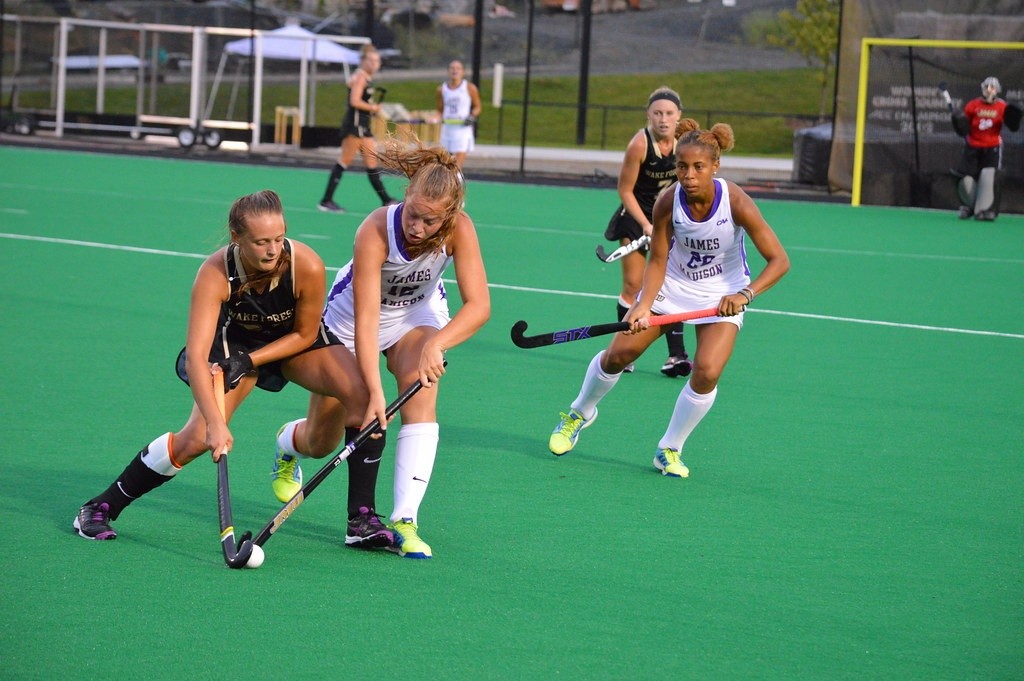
[204,25,359,125]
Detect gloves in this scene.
[218,351,255,393]
[466,115,474,125]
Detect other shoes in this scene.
[978,211,995,221]
[318,199,344,212]
[958,204,975,219]
[384,198,398,207]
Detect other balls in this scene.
[246,544,265,570]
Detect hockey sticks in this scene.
[511,306,744,350]
[239,359,448,566]
[595,227,652,266]
[210,364,254,570]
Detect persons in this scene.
[71,190,394,551]
[952,76,1024,221]
[549,118,790,477]
[604,87,693,378]
[271,145,491,559]
[431,60,481,173]
[317,44,394,212]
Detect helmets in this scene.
[980,76,1001,97]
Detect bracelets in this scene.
[737,287,755,304]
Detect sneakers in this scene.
[73,499,117,540]
[652,448,689,480]
[271,421,304,502]
[661,354,693,376]
[625,362,634,373]
[548,404,599,457]
[343,502,392,548]
[384,517,433,558]
